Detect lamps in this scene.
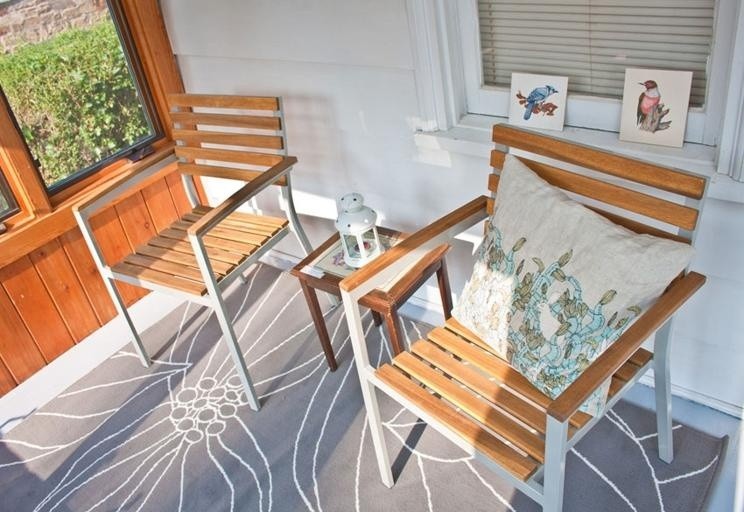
[334,192,386,268]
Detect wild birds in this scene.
[637,80,661,127]
[523,85,558,120]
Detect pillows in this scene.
[450,152,694,419]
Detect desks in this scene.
[288,224,462,381]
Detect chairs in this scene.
[337,119,709,512]
[71,93,339,412]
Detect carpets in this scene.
[0,263,730,512]
